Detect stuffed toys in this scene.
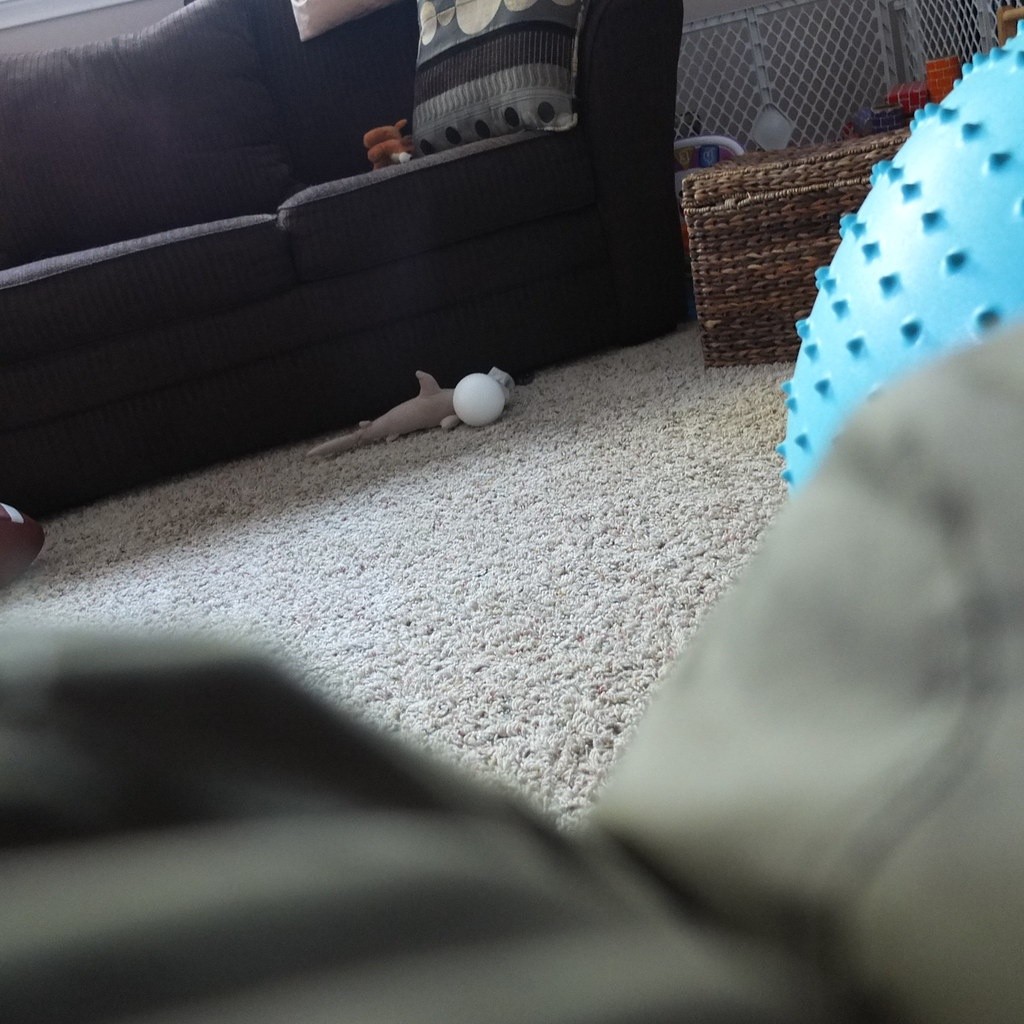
[363,118,415,171]
[302,365,517,461]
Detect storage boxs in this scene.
[870,56,962,134]
[681,127,913,370]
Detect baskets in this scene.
[680,126,917,376]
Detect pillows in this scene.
[290,0,401,43]
[410,0,589,158]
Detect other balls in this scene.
[774,21,1024,499]
[452,372,505,426]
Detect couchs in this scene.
[0,0,694,527]
